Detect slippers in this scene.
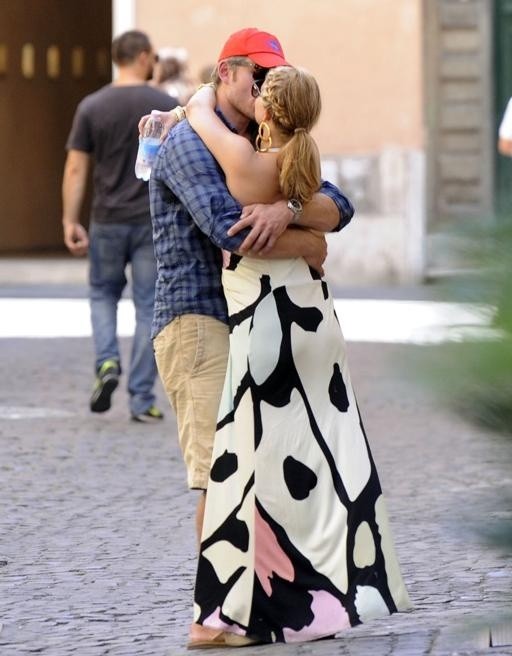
[184,628,253,651]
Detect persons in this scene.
[497,94,512,159]
[147,48,194,102]
[137,67,416,644]
[200,63,217,84]
[148,30,354,651]
[61,31,184,422]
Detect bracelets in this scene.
[171,105,186,122]
[198,84,216,91]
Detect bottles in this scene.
[135,109,166,186]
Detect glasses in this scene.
[251,80,264,96]
[230,61,268,78]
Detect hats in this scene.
[217,27,295,68]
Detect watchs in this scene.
[286,197,303,225]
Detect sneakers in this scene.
[133,406,163,423]
[90,360,120,413]
